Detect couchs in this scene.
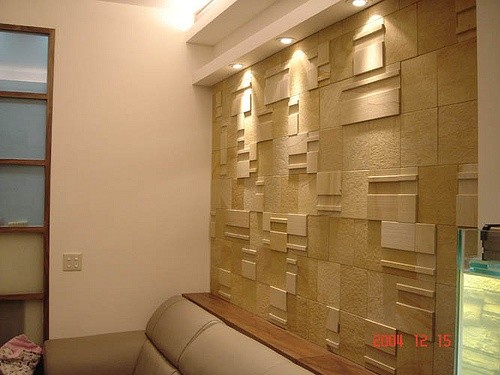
[42,293,317,375]
